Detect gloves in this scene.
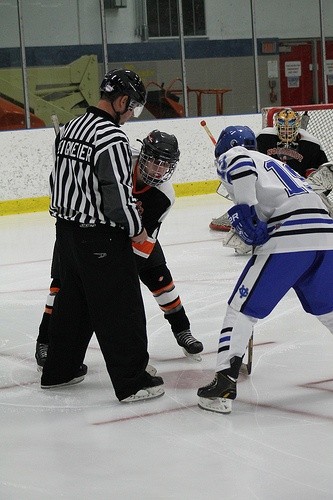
[227,204,268,245]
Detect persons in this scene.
[197,108,333,400]
[35,129,204,366]
[41,67,164,401]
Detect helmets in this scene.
[137,129,180,187]
[215,125,257,158]
[99,68,147,111]
[275,108,300,142]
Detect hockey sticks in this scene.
[51,114,60,138]
[199,119,217,146]
[238,244,258,376]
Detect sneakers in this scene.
[119,377,165,402]
[35,342,48,373]
[198,356,242,414]
[41,364,88,390]
[173,329,203,361]
